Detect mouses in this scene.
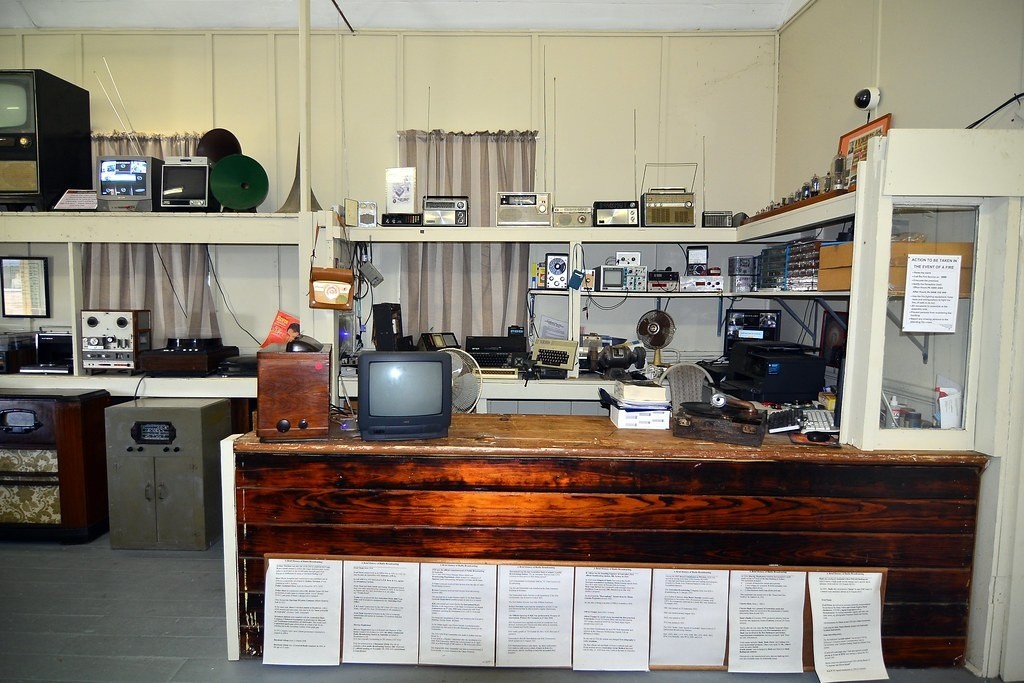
[807,431,830,442]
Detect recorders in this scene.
[640,162,698,227]
[702,210,732,227]
[553,206,593,227]
[496,191,552,227]
[592,201,639,227]
[422,195,469,227]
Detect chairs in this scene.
[658,363,715,419]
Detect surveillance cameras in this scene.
[853,87,880,110]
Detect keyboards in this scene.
[801,409,840,434]
[536,348,569,366]
[469,354,509,367]
[768,408,804,433]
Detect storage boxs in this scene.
[610,404,670,430]
[815,242,974,294]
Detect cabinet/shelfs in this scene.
[0,211,298,398]
[105,397,231,553]
[339,191,856,296]
[0,388,111,544]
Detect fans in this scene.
[637,310,675,375]
[436,348,483,416]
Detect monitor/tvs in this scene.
[723,309,782,357]
[0,75,35,133]
[160,164,213,208]
[96,155,165,212]
[834,357,846,427]
[358,350,452,441]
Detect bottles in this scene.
[756,151,846,215]
[886,395,922,430]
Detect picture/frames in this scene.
[0,256,51,319]
[837,112,893,195]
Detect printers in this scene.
[707,340,827,405]
